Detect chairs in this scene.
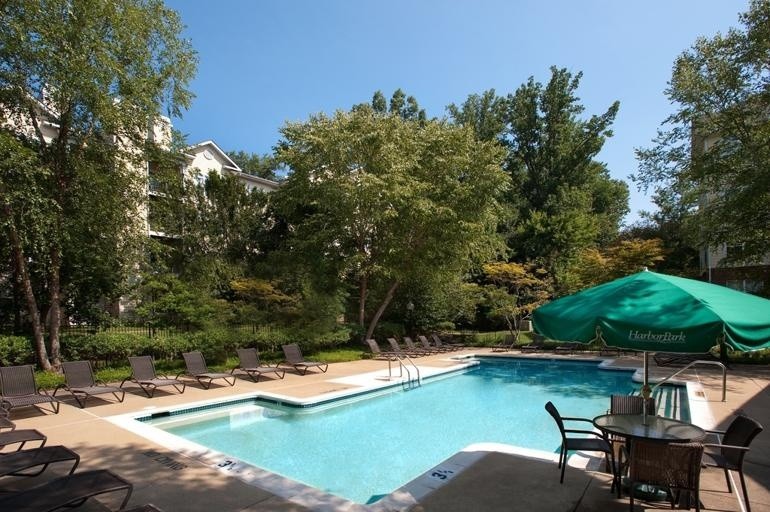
[53,360,125,409]
[0,446,80,490]
[0,404,16,432]
[117,504,163,512]
[365,334,465,361]
[0,429,47,454]
[174,351,237,389]
[120,356,186,398]
[490,332,638,357]
[276,343,329,376]
[1,469,134,512]
[230,347,285,383]
[653,351,729,368]
[2,365,59,416]
[544,394,764,512]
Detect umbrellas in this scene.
[530,265,769,502]
